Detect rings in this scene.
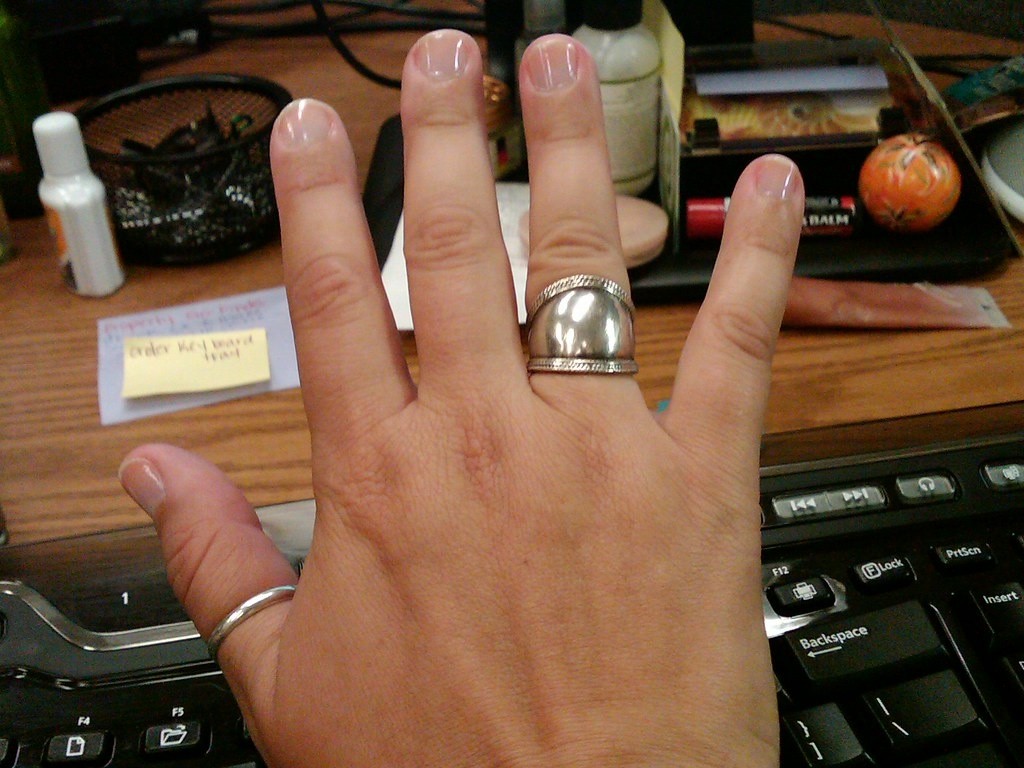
[526,273,640,375]
[207,584,296,664]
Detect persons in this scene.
[119,28,805,768]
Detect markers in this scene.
[684,191,862,240]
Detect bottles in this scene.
[31,110,126,298]
[514,0,567,115]
[570,0,660,197]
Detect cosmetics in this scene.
[33,111,124,297]
[783,278,1010,332]
[485,0,869,271]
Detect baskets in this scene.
[74,69,293,268]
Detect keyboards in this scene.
[0,430,1024,768]
